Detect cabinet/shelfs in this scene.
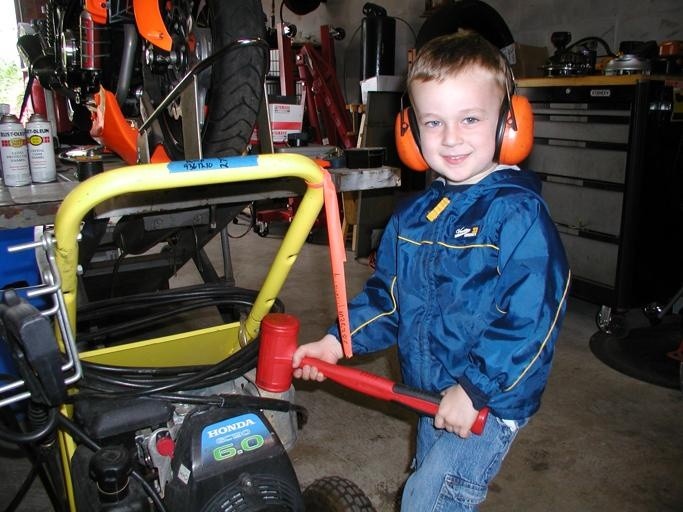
[499,76,680,313]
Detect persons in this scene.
[289,29,572,511]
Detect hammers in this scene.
[256,313,490,435]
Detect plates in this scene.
[55,146,120,164]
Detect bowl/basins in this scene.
[342,147,385,169]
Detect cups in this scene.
[287,133,309,147]
[77,160,104,182]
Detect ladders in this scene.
[296,44,356,149]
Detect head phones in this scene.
[394,32,535,171]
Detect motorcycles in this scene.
[16,2,275,165]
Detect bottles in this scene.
[0,111,58,187]
